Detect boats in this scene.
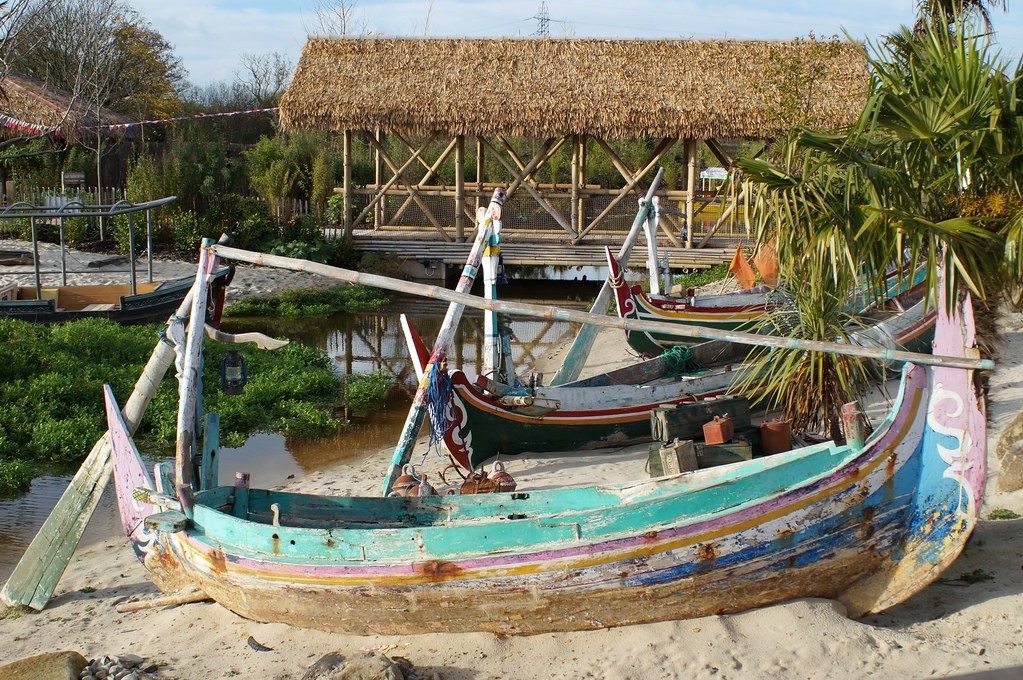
[0,196,241,330]
[100,195,997,631]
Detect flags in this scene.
[729,244,757,290]
[754,240,777,285]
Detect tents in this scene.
[700,166,727,197]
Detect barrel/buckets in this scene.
[41,289,59,308]
[761,422,790,452]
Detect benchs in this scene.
[82,303,115,311]
